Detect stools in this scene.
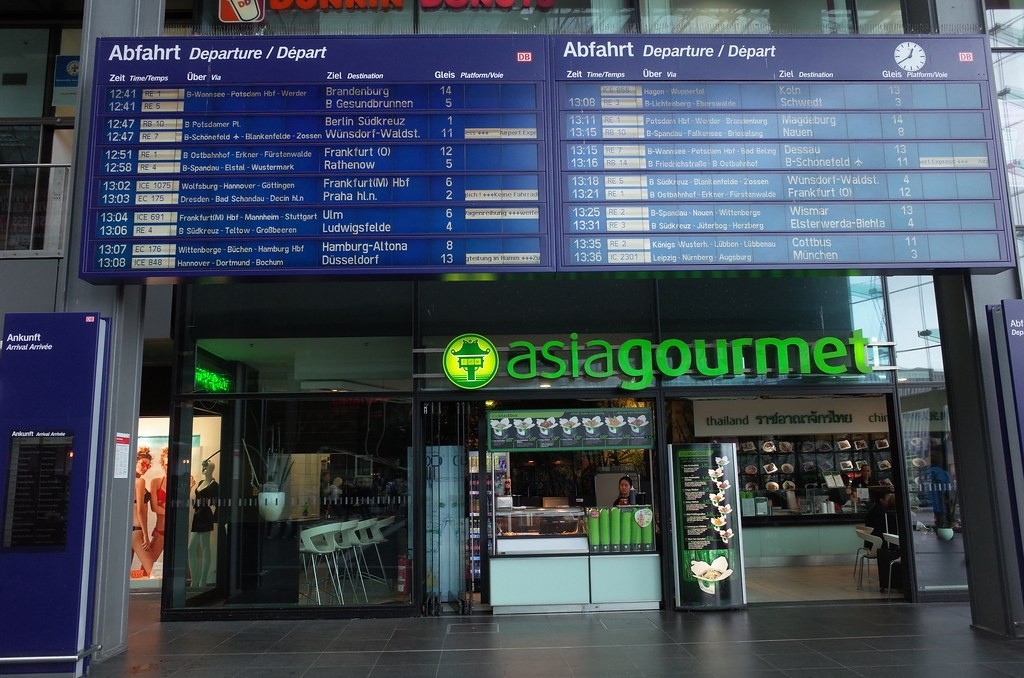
[297,516,395,605]
[850,525,902,596]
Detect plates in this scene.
[738,439,925,490]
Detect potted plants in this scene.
[254,448,295,521]
[929,491,958,541]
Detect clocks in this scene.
[894,42,926,71]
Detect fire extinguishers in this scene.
[397,547,412,593]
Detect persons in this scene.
[612,476,637,506]
[850,458,882,508]
[864,487,900,594]
[131,448,168,578]
[188,460,220,588]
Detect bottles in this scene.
[467,457,510,592]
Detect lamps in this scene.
[202,450,220,471]
